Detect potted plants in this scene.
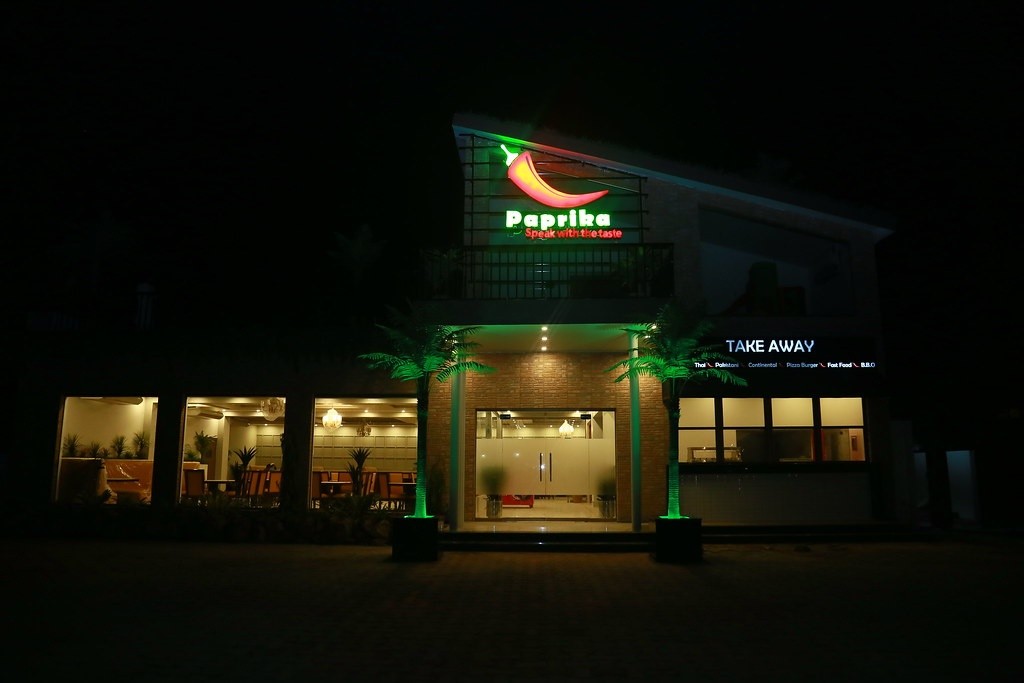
[356,296,494,562]
[596,495,614,520]
[485,495,502,519]
[604,285,749,565]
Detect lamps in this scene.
[322,406,342,432]
[260,397,285,421]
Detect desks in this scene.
[388,482,416,513]
[107,478,139,483]
[203,480,236,491]
[320,481,352,495]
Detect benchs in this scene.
[379,497,411,512]
